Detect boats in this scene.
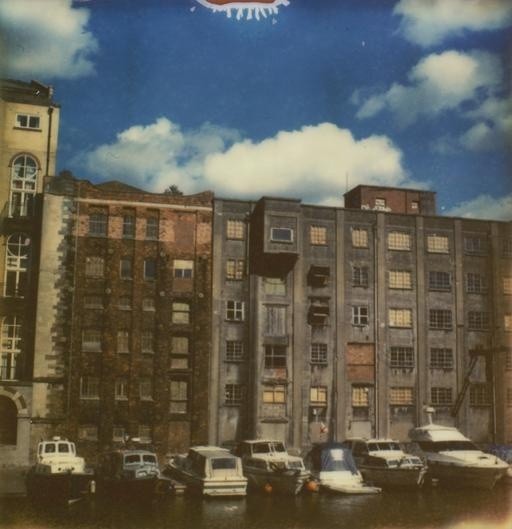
[299,441,381,494]
[405,424,509,491]
[233,440,310,495]
[34,437,247,506]
[344,436,426,487]
